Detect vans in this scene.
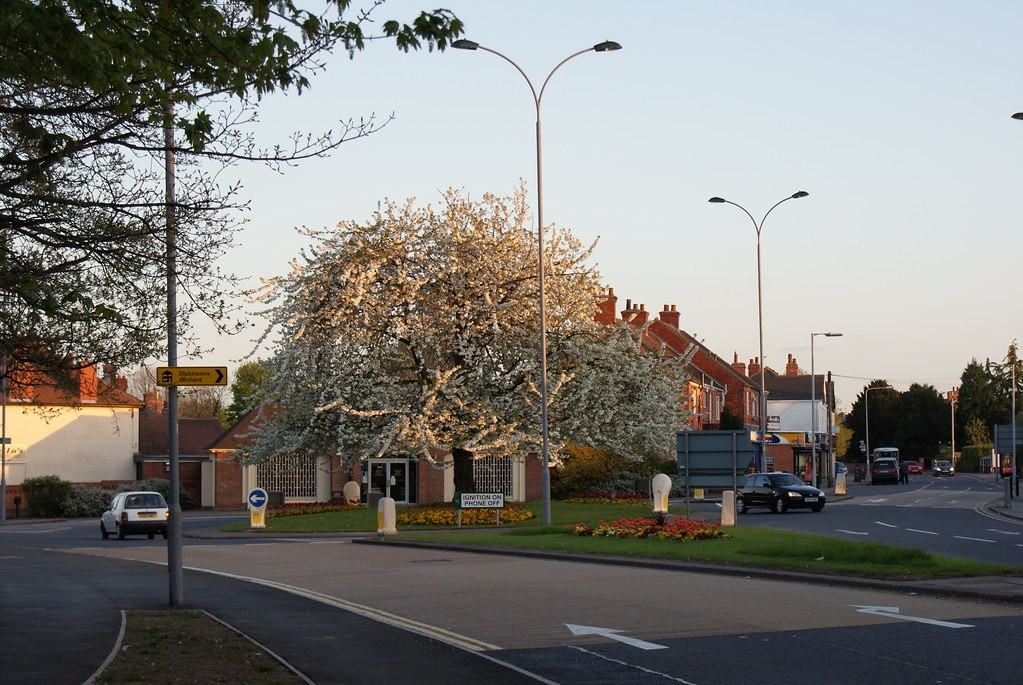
[873,448,900,465]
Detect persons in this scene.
[898,459,910,484]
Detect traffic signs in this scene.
[157,366,228,386]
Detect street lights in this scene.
[989,363,1017,499]
[708,191,809,472]
[451,41,625,527]
[810,331,844,487]
[865,385,894,484]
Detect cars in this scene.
[835,462,848,479]
[904,460,924,476]
[736,472,826,515]
[872,459,900,485]
[99,491,169,541]
[932,460,955,477]
